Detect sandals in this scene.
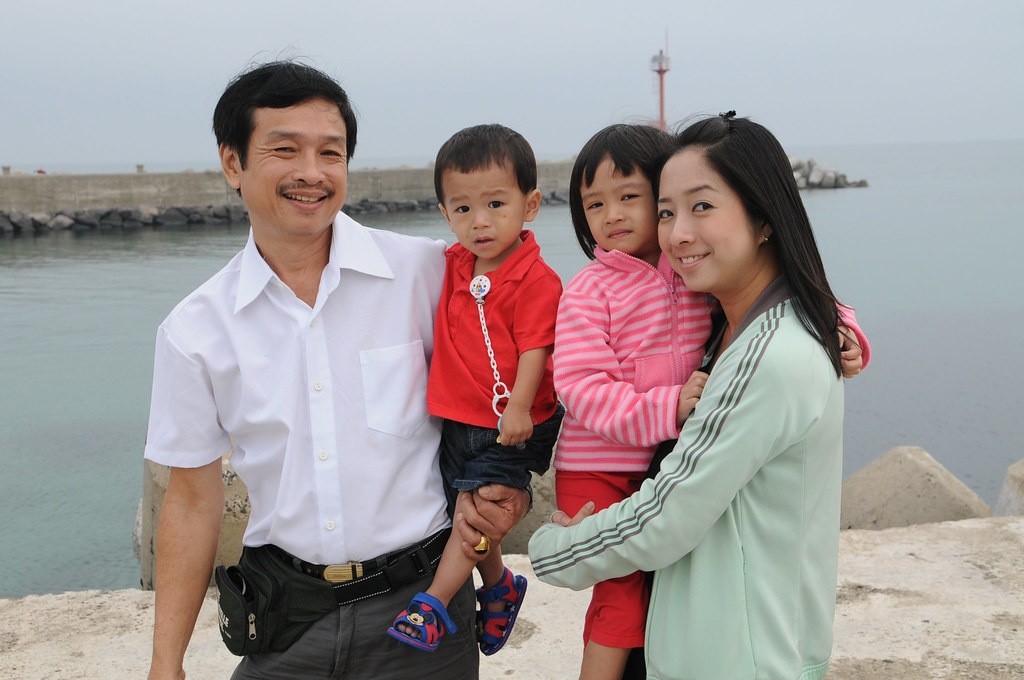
[386,591,457,653]
[473,566,527,656]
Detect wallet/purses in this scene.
[213,563,286,656]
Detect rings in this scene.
[472,534,491,556]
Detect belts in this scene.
[261,528,445,586]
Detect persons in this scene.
[551,121,871,668]
[524,110,846,680]
[384,125,565,653]
[137,60,538,679]
